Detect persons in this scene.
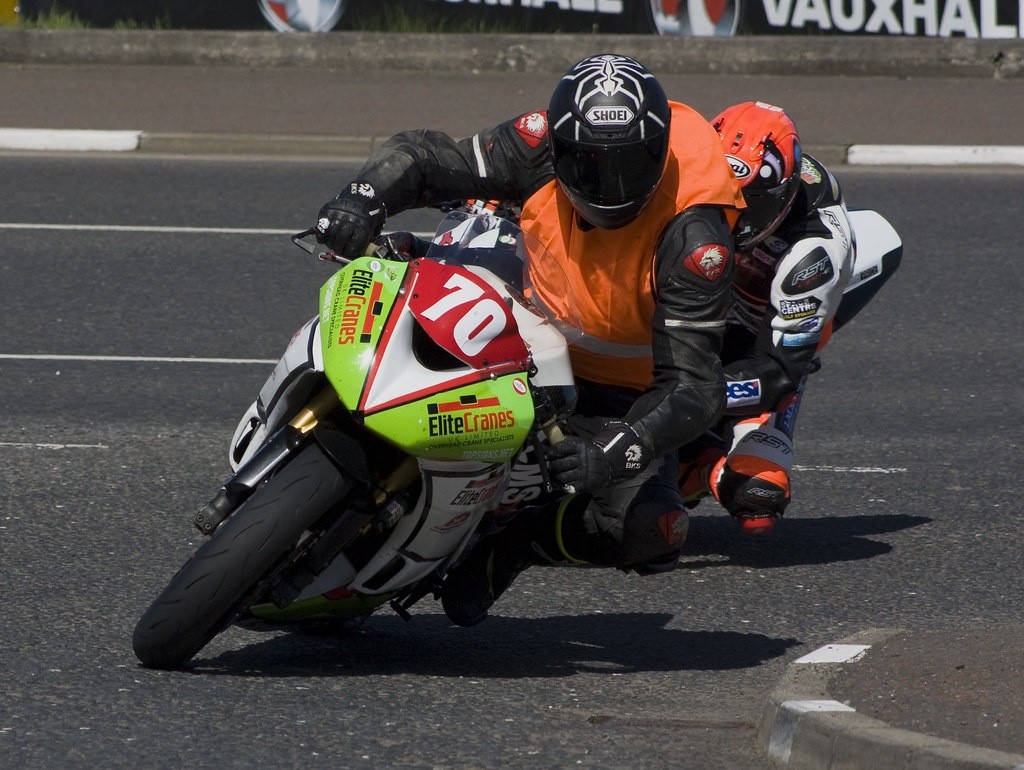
[678,103,856,536]
[316,53,747,625]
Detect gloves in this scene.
[315,178,390,258]
[546,422,645,495]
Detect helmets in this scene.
[709,101,803,254]
[550,54,672,231]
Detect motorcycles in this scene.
[132,210,903,670]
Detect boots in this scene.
[443,494,591,628]
[676,448,725,507]
